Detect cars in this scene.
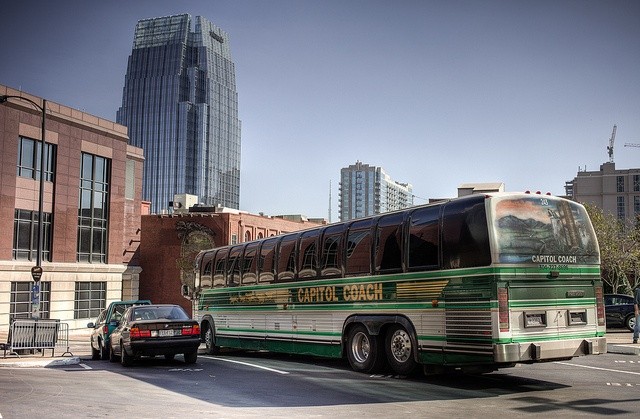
[604,294,639,331]
[108,303,202,366]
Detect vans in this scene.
[87,299,151,359]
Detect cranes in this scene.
[603,118,634,170]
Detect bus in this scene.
[181,190,607,372]
